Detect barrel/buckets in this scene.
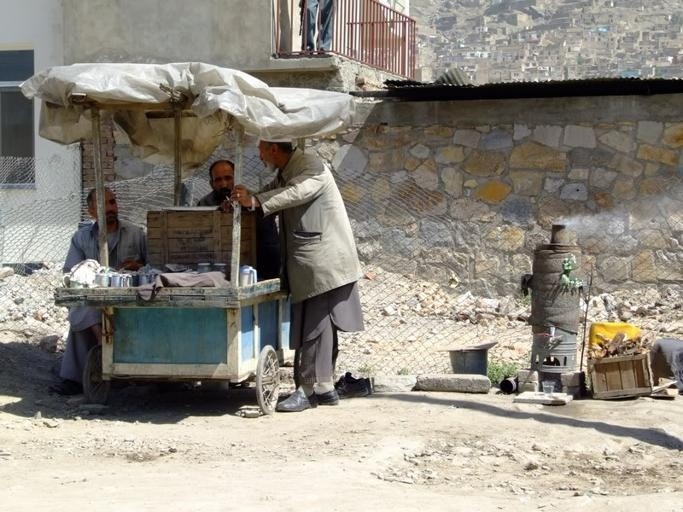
[449,349,487,376]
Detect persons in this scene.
[48,183,148,397]
[228,129,367,415]
[298,0,336,58]
[196,159,283,288]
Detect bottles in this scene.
[197,261,229,281]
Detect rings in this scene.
[236,192,243,199]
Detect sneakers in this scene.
[49,380,82,396]
[278,390,339,411]
[335,372,373,397]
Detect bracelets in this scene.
[250,195,257,212]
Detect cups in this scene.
[237,265,257,287]
[542,380,555,397]
[95,270,133,288]
[63,274,87,288]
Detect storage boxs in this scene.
[146,209,258,279]
[587,346,653,399]
